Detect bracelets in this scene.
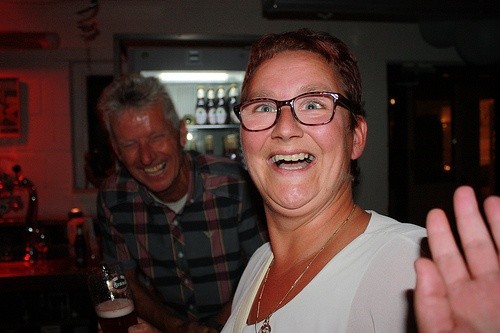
[208,318,223,332]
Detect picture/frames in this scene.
[0,77,23,139]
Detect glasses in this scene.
[234,90,360,132]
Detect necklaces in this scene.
[255,202,357,333]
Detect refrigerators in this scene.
[139,69,244,168]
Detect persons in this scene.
[95,74,265,333]
[97,30,500,333]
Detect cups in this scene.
[87,263,139,333]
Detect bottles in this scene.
[223,134,240,162]
[204,134,214,155]
[194,84,239,125]
[74,224,88,268]
